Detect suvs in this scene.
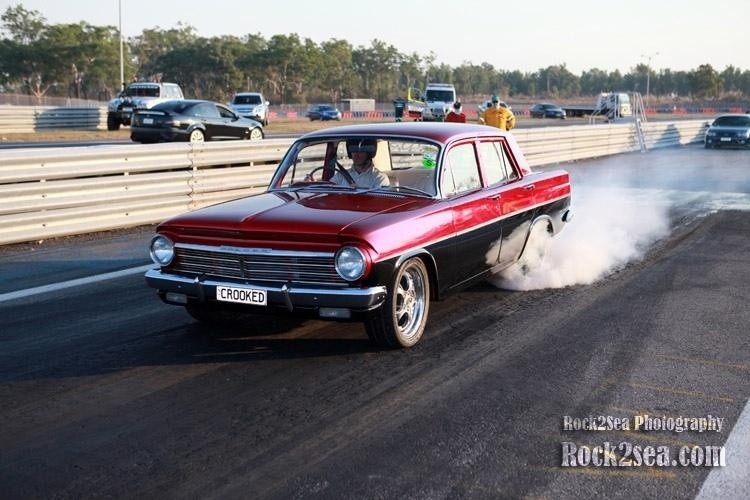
[107,80,185,131]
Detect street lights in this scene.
[641,51,660,109]
[546,66,553,99]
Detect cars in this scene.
[143,121,573,350]
[704,114,750,149]
[130,99,264,144]
[528,103,566,120]
[478,100,512,125]
[305,104,342,122]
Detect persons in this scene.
[444,100,468,123]
[392,97,405,122]
[301,137,392,191]
[477,95,516,131]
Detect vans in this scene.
[226,92,270,126]
[407,83,462,122]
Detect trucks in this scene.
[561,91,632,119]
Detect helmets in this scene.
[346,138,377,159]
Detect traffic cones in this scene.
[672,105,678,115]
